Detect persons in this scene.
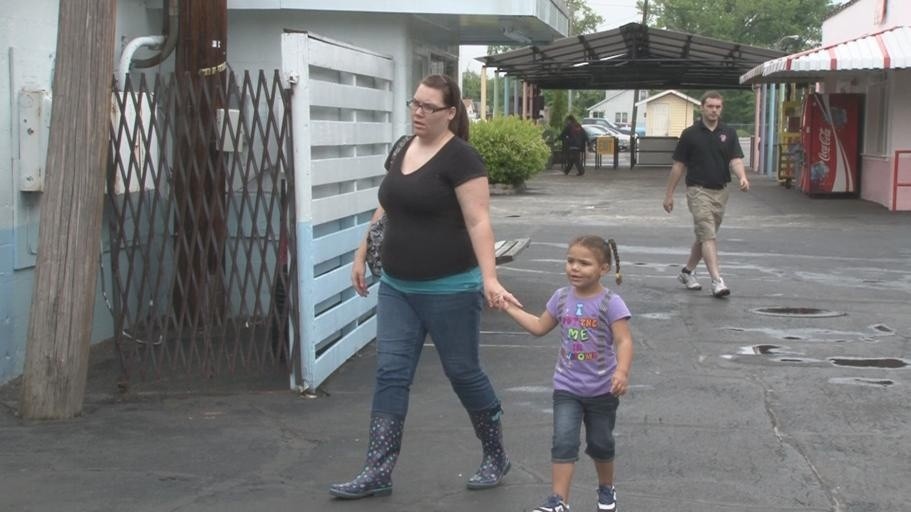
[557,114,591,176]
[497,235,637,511]
[328,72,524,499]
[663,90,755,295]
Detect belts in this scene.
[688,180,727,190]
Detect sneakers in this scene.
[596,485,617,512]
[677,270,701,290]
[711,278,730,297]
[532,492,570,512]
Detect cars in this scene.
[582,123,637,152]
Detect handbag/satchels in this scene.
[366,135,414,278]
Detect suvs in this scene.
[584,117,639,139]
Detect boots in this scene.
[329,410,406,500]
[467,399,511,489]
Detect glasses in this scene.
[406,100,450,114]
